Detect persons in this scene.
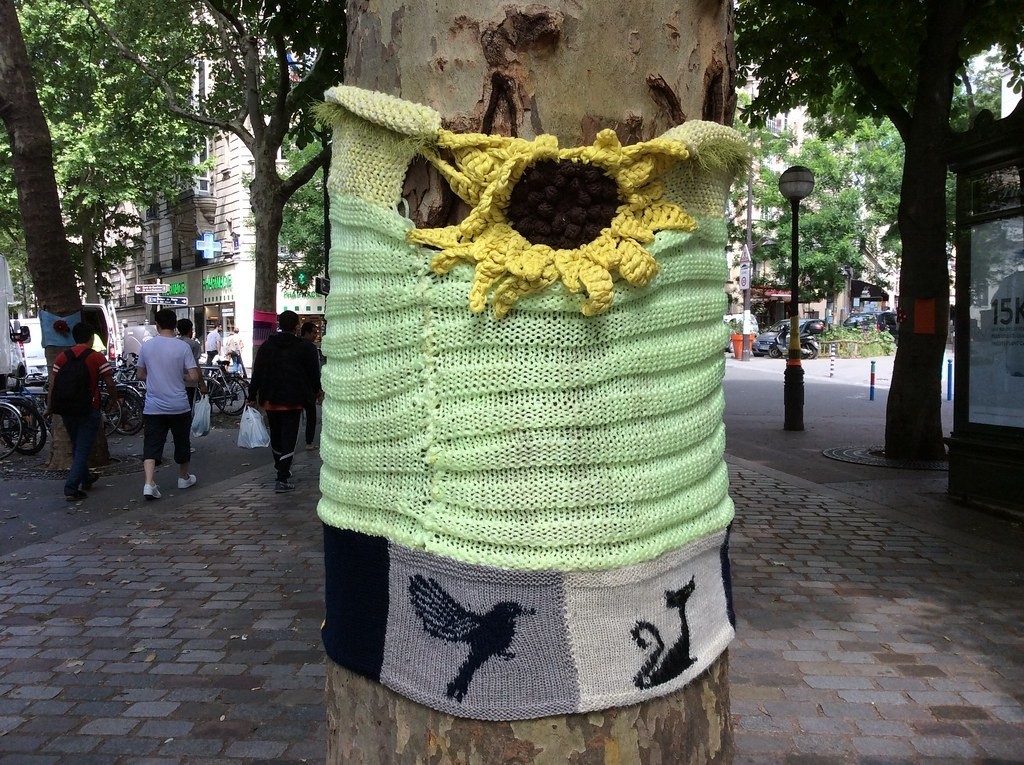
[300,323,320,451]
[204,324,222,375]
[42,322,118,501]
[136,309,202,498]
[246,310,322,493]
[192,332,200,344]
[215,328,248,378]
[175,318,207,454]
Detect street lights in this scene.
[778,165,814,432]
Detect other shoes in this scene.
[190,448,196,452]
[64,490,88,501]
[304,442,319,450]
[82,473,99,490]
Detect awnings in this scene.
[851,280,889,301]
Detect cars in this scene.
[751,318,832,358]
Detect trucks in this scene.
[0,255,160,391]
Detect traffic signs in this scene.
[144,295,189,307]
[135,284,170,294]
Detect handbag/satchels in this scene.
[211,352,220,366]
[191,394,211,438]
[237,404,270,449]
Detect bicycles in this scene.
[0,352,250,462]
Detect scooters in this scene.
[768,323,819,360]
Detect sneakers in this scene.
[143,484,162,498]
[274,481,295,492]
[178,474,196,488]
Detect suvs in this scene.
[723,313,758,353]
[842,312,896,335]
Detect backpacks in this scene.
[50,348,95,415]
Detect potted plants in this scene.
[730,320,755,358]
